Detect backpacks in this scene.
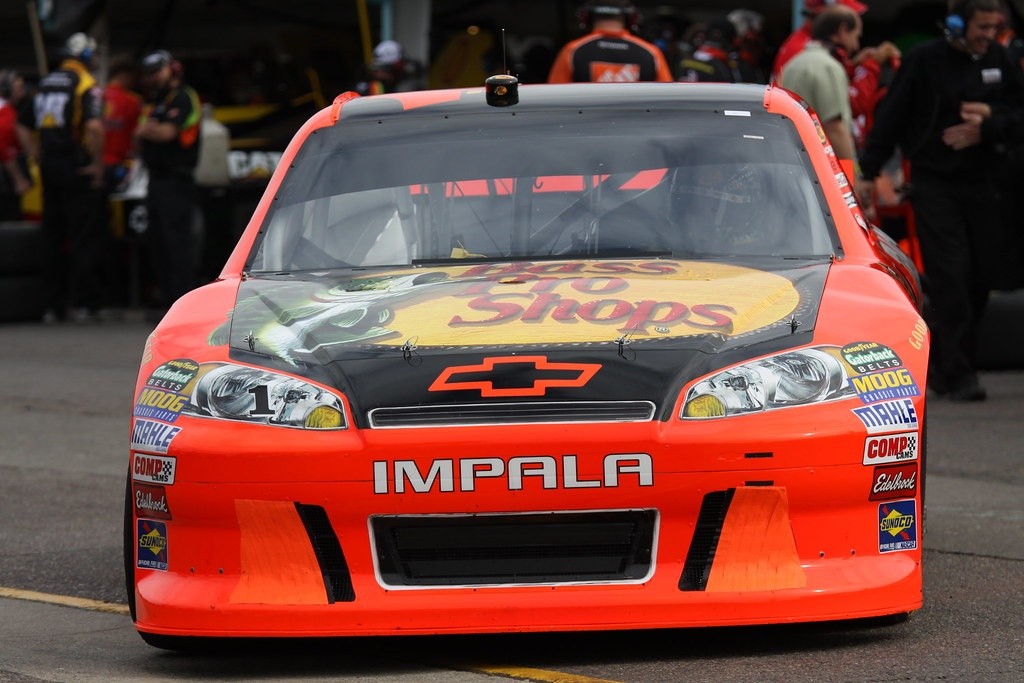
[32,70,81,132]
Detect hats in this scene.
[67,33,96,57]
[372,40,402,67]
[805,0,868,16]
[141,50,171,73]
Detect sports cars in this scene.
[124,27,932,651]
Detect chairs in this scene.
[291,167,418,269]
[664,165,770,270]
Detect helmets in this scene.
[663,146,771,253]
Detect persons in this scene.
[771,2,902,196]
[890,0,1024,403]
[657,0,764,84]
[136,51,199,321]
[34,33,101,324]
[546,0,673,83]
[371,40,421,94]
[0,67,35,222]
[102,61,142,321]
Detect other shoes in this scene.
[928,367,989,402]
[43,308,147,323]
